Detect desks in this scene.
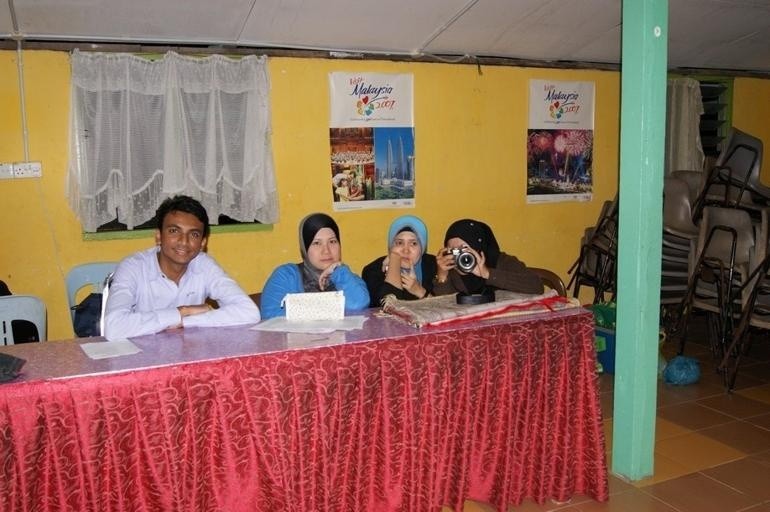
[1,288,605,507]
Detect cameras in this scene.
[443,244,477,272]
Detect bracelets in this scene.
[422,289,431,298]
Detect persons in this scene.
[432,218,544,295]
[103,195,261,341]
[259,213,371,320]
[332,168,367,201]
[362,214,436,308]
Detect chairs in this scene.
[500,255,570,301]
[62,264,117,336]
[579,128,767,385]
[1,293,48,348]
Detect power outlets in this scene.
[14,163,42,178]
